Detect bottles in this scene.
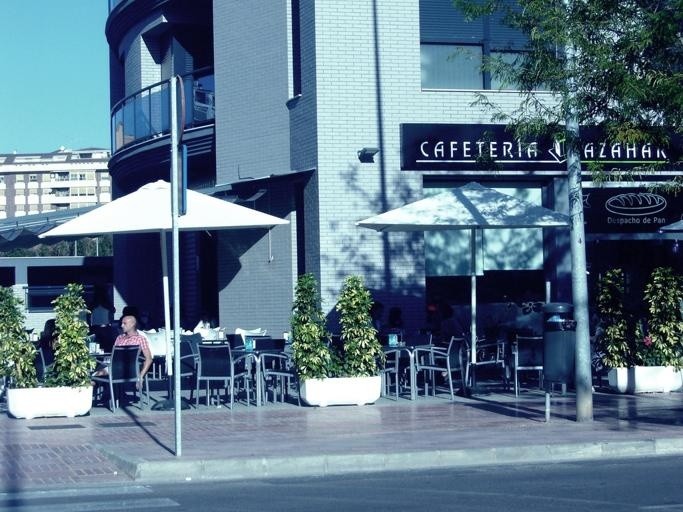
[218,329,225,339]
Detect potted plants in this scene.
[291,271,387,407]
[598,266,682,392]
[0,282,97,420]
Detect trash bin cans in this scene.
[543,302,577,383]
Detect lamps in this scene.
[359,148,380,159]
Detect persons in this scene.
[371,302,385,329]
[93,314,152,390]
[382,307,415,347]
[40,320,59,353]
[438,305,462,338]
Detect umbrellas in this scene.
[35,178,292,398]
[356,181,587,387]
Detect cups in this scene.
[88,342,96,354]
[282,332,289,342]
[99,349,104,354]
[95,344,100,354]
[244,336,253,351]
[388,332,398,347]
